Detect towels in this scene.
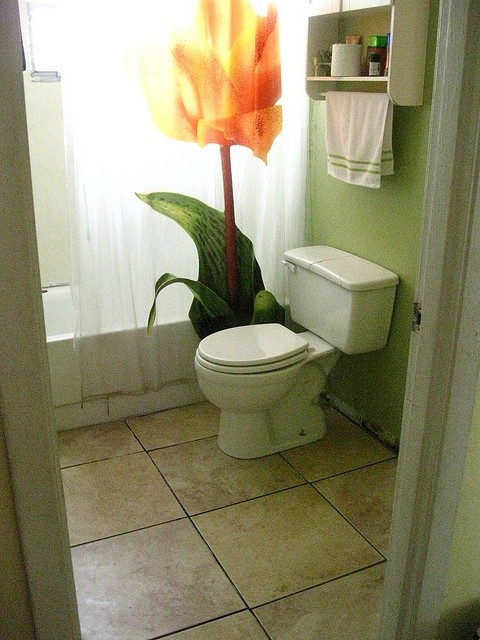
[326,89,395,189]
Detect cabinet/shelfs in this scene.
[306,2,429,106]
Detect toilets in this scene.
[193,245,399,459]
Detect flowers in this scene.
[136,2,286,341]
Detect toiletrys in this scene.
[384,33,391,76]
[365,34,387,75]
